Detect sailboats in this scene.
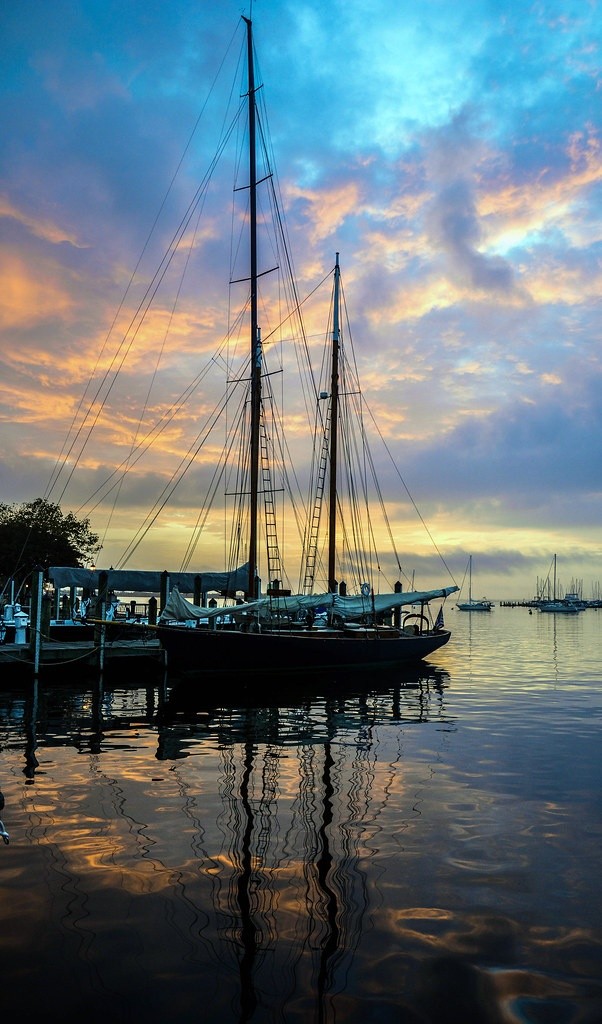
[531,554,602,613]
[0,1,462,675]
[455,554,495,611]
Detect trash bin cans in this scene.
[3,603,14,621]
